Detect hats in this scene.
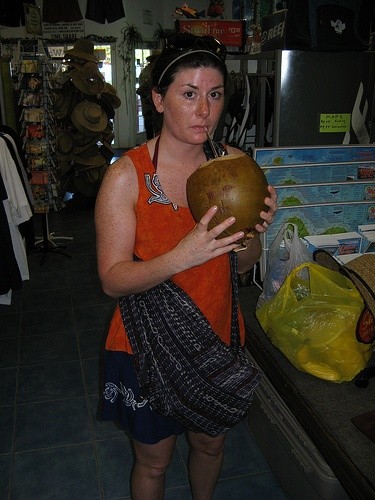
[313,248,375,344]
[71,102,108,134]
[67,69,104,97]
[98,133,116,156]
[75,146,105,181]
[64,36,100,63]
[71,130,98,156]
[101,81,122,109]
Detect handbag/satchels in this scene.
[112,256,263,438]
[256,222,374,383]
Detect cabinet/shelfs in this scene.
[210,49,375,160]
